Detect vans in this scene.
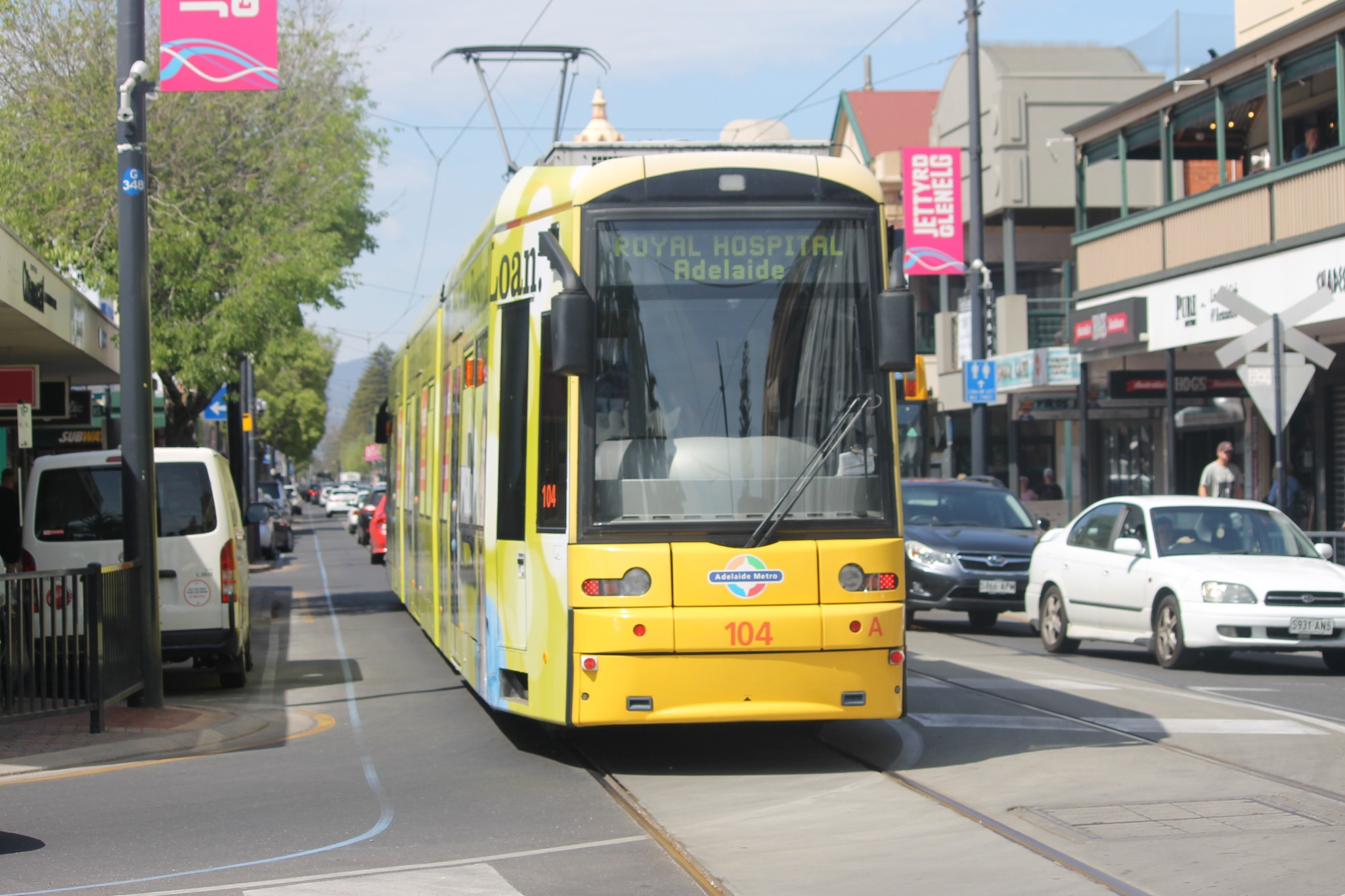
[19,445,252,691]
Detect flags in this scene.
[159,0,280,91]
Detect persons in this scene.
[1198,440,1244,499]
[1020,467,1063,501]
[1292,125,1328,160]
[1144,516,1195,551]
[1263,460,1302,512]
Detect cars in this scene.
[902,475,1051,633]
[1024,493,1345,674]
[249,472,388,566]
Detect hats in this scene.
[1218,440,1234,453]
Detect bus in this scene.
[373,45,918,730]
